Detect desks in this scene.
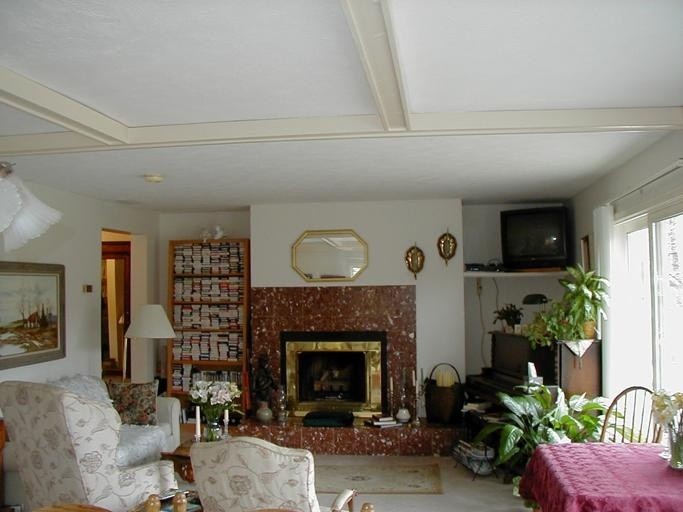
[519,440,682,511]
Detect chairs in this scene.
[0,380,175,512]
[189,434,371,512]
[593,385,665,442]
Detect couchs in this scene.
[0,369,182,489]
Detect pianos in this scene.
[465,331,601,472]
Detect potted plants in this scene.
[560,266,598,339]
[251,350,276,423]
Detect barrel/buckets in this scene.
[423,363,462,427]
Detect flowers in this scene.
[188,380,244,418]
[653,388,682,429]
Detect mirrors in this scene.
[290,228,368,282]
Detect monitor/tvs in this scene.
[499,205,569,271]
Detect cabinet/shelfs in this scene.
[165,235,249,398]
[467,334,597,398]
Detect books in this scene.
[452,439,496,475]
[460,401,505,425]
[371,413,393,421]
[169,240,243,394]
[371,417,396,425]
[364,420,403,429]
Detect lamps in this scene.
[121,301,175,378]
[0,158,62,257]
[389,375,393,395]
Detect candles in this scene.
[412,369,415,386]
[194,404,200,437]
[222,401,229,423]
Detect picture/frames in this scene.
[0,260,66,373]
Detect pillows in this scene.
[47,372,111,404]
[107,382,158,428]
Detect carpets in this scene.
[312,462,444,493]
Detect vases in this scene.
[395,410,410,423]
[205,421,219,441]
[665,429,681,467]
